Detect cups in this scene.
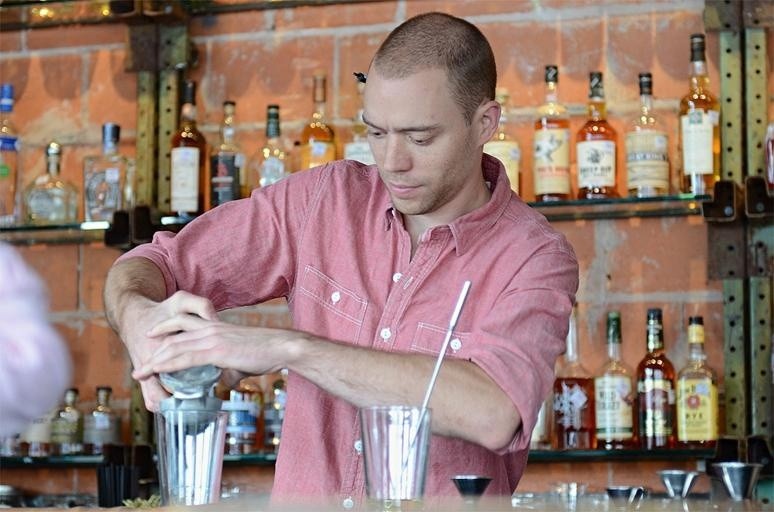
[654,470,706,499]
[708,460,763,501]
[608,486,646,501]
[358,404,431,502]
[451,474,493,499]
[155,410,224,503]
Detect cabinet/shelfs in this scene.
[184,0,726,469]
[0,15,137,469]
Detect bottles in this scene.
[678,33,722,200]
[624,72,674,202]
[0,370,288,457]
[525,300,722,450]
[488,86,523,200]
[575,70,619,203]
[1,87,135,229]
[164,71,377,235]
[535,65,572,203]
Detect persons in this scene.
[3,243,75,444]
[97,12,583,502]
